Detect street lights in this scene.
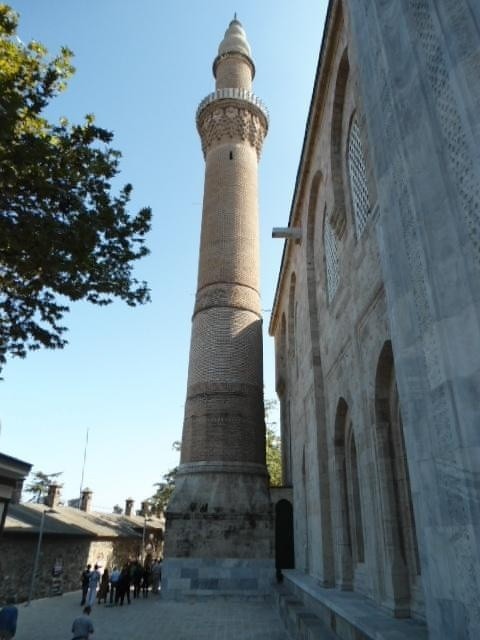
[25,509,58,607]
[140,518,153,565]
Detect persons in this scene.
[0,598,17,640]
[72,607,93,640]
[81,555,162,607]
